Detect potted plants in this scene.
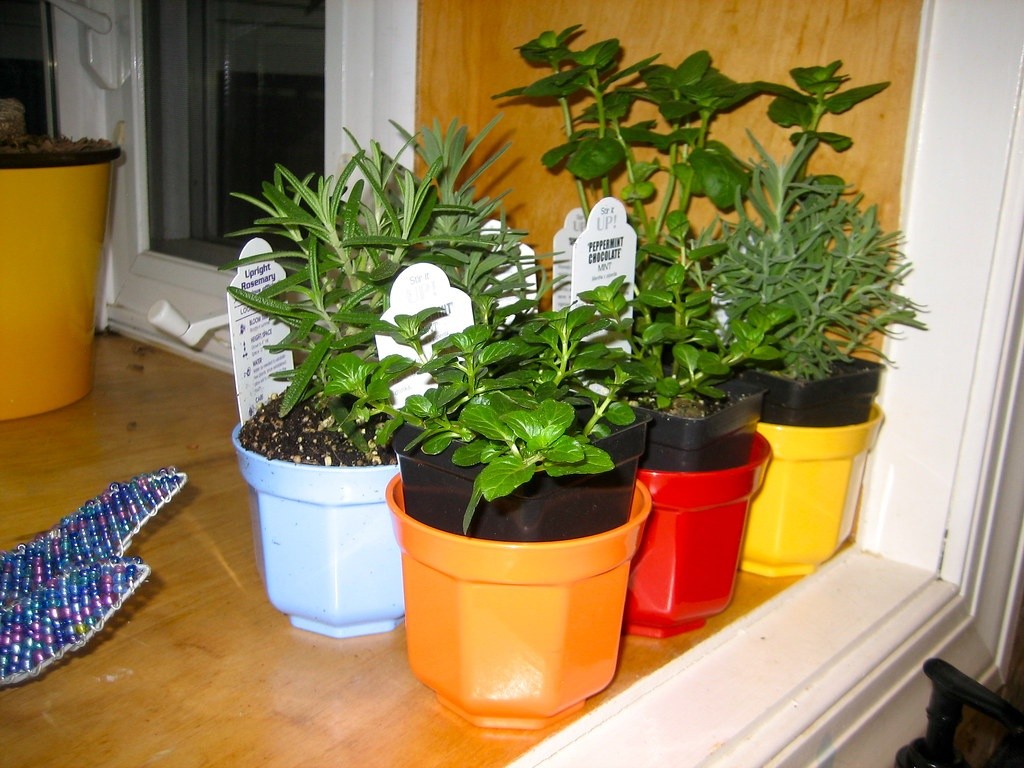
[0,0,122,421]
[699,125,930,579]
[575,207,771,637]
[324,295,651,731]
[216,126,439,636]
